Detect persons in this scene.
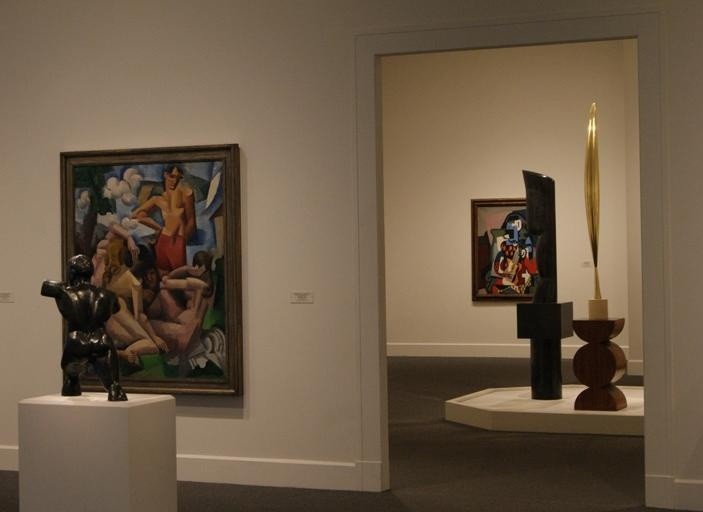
[40,253,128,402]
[89,162,216,368]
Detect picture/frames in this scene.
[59,142,245,399]
[469,198,536,302]
